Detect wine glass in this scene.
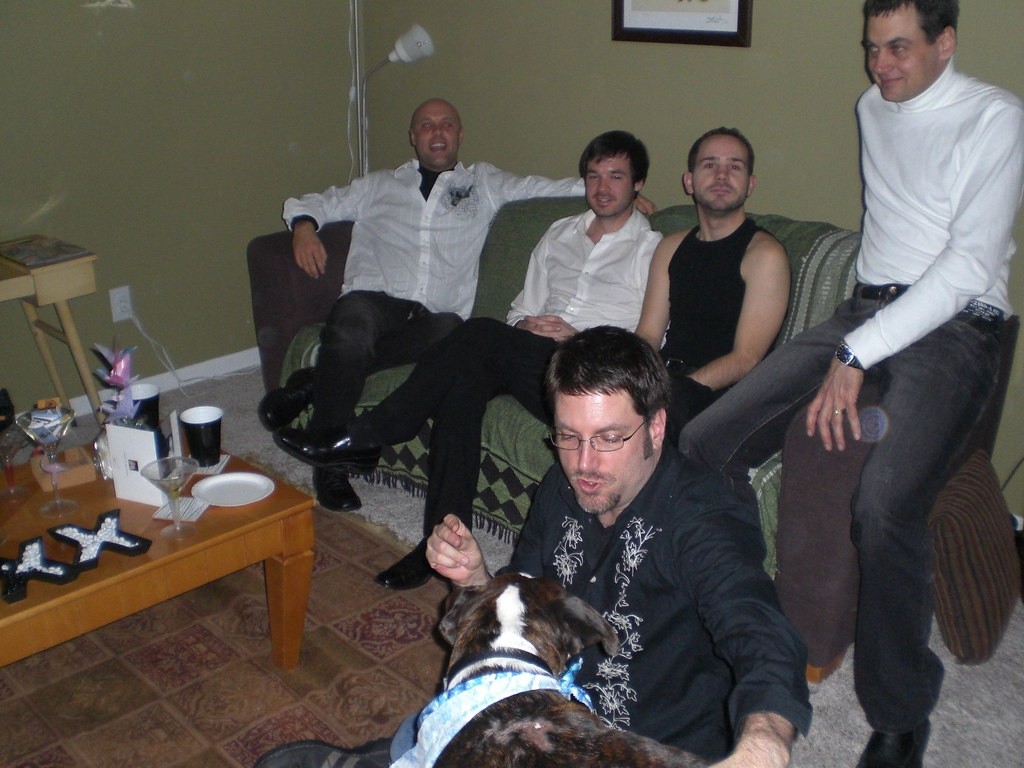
[140,456,199,540]
[0,423,27,505]
[14,406,82,519]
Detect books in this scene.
[0,233,88,267]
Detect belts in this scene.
[659,357,701,377]
[851,283,1003,333]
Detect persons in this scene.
[270,129,665,588]
[679,0,1023,768]
[256,326,813,768]
[512,127,790,593]
[257,97,656,511]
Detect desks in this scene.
[0,237,108,425]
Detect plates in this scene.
[191,471,275,506]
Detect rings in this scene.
[556,327,560,332]
[832,410,843,414]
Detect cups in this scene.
[179,406,223,468]
[130,383,161,430]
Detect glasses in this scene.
[548,419,650,451]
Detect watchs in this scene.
[834,339,863,371]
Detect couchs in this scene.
[248,191,1016,678]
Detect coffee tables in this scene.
[0,428,321,682]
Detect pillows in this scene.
[921,454,1016,664]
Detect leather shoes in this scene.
[375,537,440,589]
[271,428,383,475]
[855,718,931,768]
[313,464,363,512]
[259,367,320,433]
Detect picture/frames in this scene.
[611,0,753,47]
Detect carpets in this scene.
[0,502,451,768]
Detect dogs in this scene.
[391,567,709,768]
